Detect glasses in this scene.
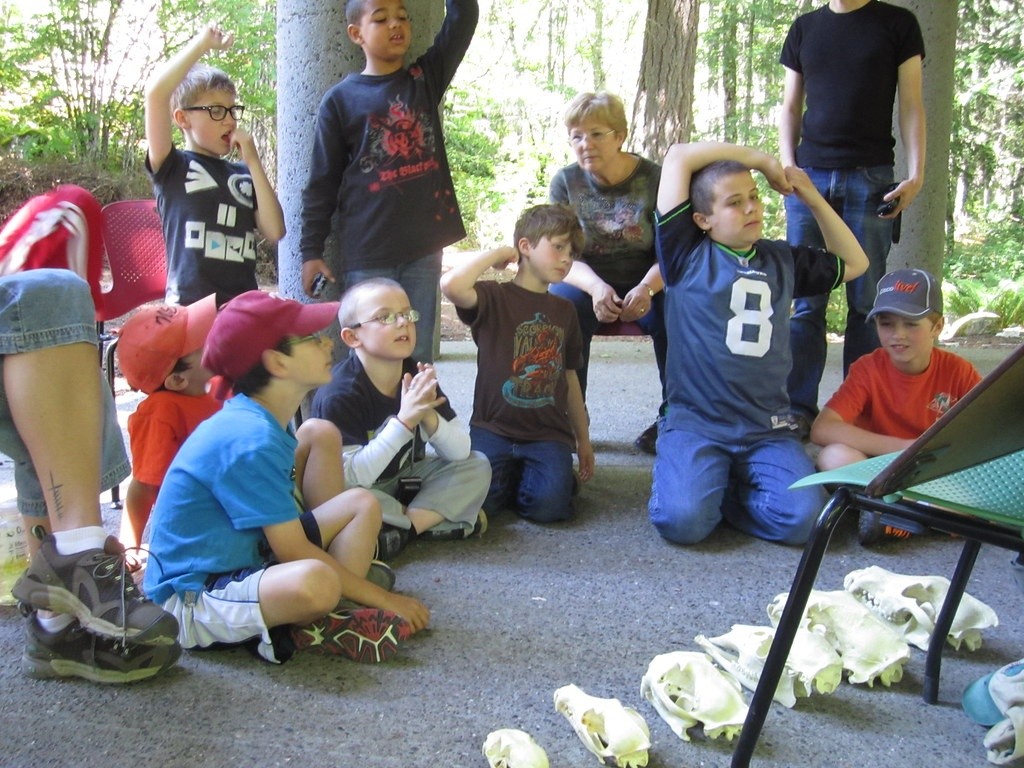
[284,325,334,348]
[348,308,419,331]
[177,106,247,121]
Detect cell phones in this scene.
[311,274,327,298]
[877,182,900,216]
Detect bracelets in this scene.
[642,283,654,296]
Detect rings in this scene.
[640,308,645,313]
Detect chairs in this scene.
[86,199,166,509]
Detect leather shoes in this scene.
[634,421,657,452]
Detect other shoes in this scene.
[857,508,915,544]
[288,605,413,667]
[360,562,400,597]
[428,508,490,540]
[375,523,411,562]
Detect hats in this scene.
[200,288,341,383]
[119,289,218,394]
[865,268,944,318]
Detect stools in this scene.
[729,447,1024,768]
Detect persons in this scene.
[142,290,430,664]
[298,0,479,368]
[440,204,594,521]
[118,292,395,595]
[0,268,181,681]
[310,278,492,562]
[548,93,665,454]
[812,267,984,548]
[145,22,287,311]
[773,0,927,442]
[645,142,870,547]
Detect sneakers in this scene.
[9,524,181,647]
[16,610,185,684]
